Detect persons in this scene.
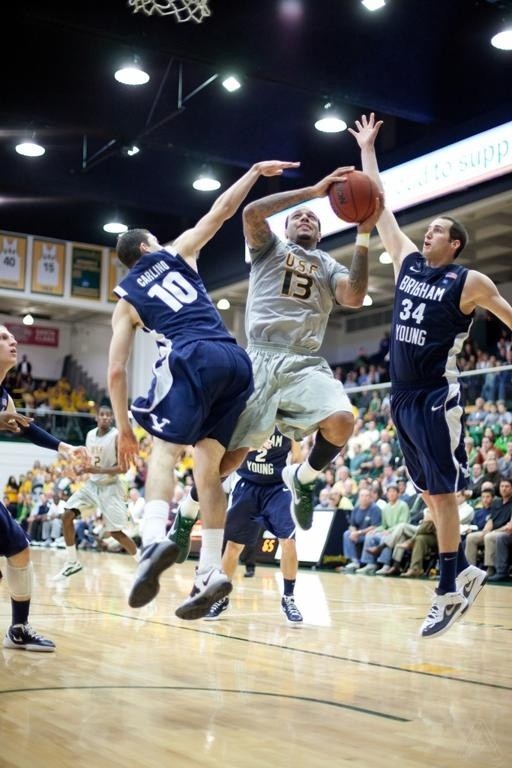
[190,164,386,531]
[347,109,512,636]
[1,310,512,580]
[105,158,304,619]
[0,320,93,652]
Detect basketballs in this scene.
[330,171,377,223]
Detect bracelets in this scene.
[355,232,371,249]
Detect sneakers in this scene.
[420,565,489,639]
[341,559,424,579]
[3,623,57,651]
[487,573,509,581]
[176,566,234,620]
[279,594,304,624]
[124,538,178,607]
[244,564,254,577]
[168,507,198,562]
[52,561,82,585]
[289,463,319,530]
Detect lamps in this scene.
[315,103,348,133]
[102,209,129,232]
[113,52,150,87]
[192,162,221,192]
[491,16,512,51]
[15,131,46,159]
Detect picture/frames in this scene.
[0,233,129,303]
